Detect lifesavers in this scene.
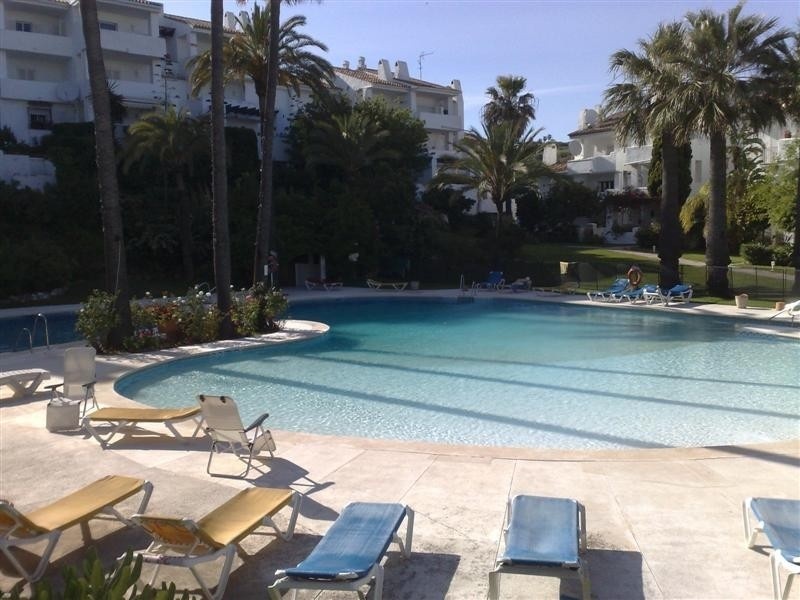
[628,269,642,286]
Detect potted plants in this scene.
[409,272,420,290]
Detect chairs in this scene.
[367,278,408,291]
[307,280,344,292]
[272,500,415,600]
[195,393,277,482]
[82,406,208,450]
[117,487,303,600]
[488,495,591,600]
[44,346,97,417]
[0,368,50,399]
[742,497,800,600]
[0,475,155,582]
[479,272,694,307]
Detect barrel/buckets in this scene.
[735,296,748,308]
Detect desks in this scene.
[45,401,81,432]
